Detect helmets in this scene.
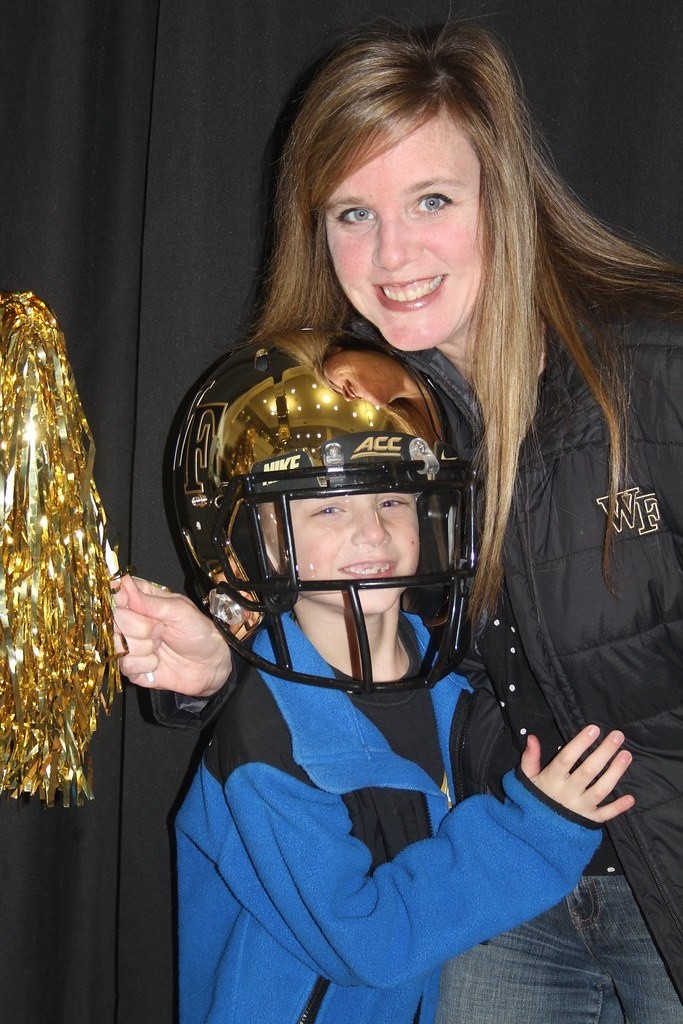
[171,332,488,696]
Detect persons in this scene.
[171,325,637,1024]
[109,21,683,1023]
